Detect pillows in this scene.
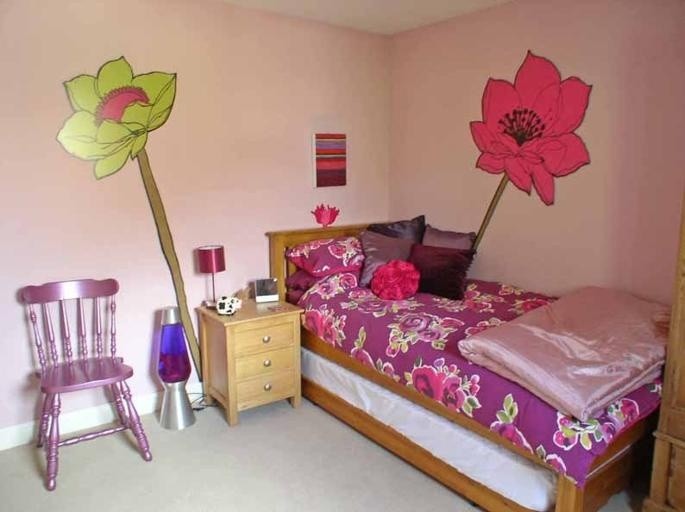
[282,213,477,296]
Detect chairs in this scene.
[19,276,153,492]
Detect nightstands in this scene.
[193,297,306,427]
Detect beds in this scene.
[262,220,667,511]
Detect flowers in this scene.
[470,48,594,254]
[309,203,340,228]
[52,56,204,385]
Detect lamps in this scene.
[196,244,226,310]
